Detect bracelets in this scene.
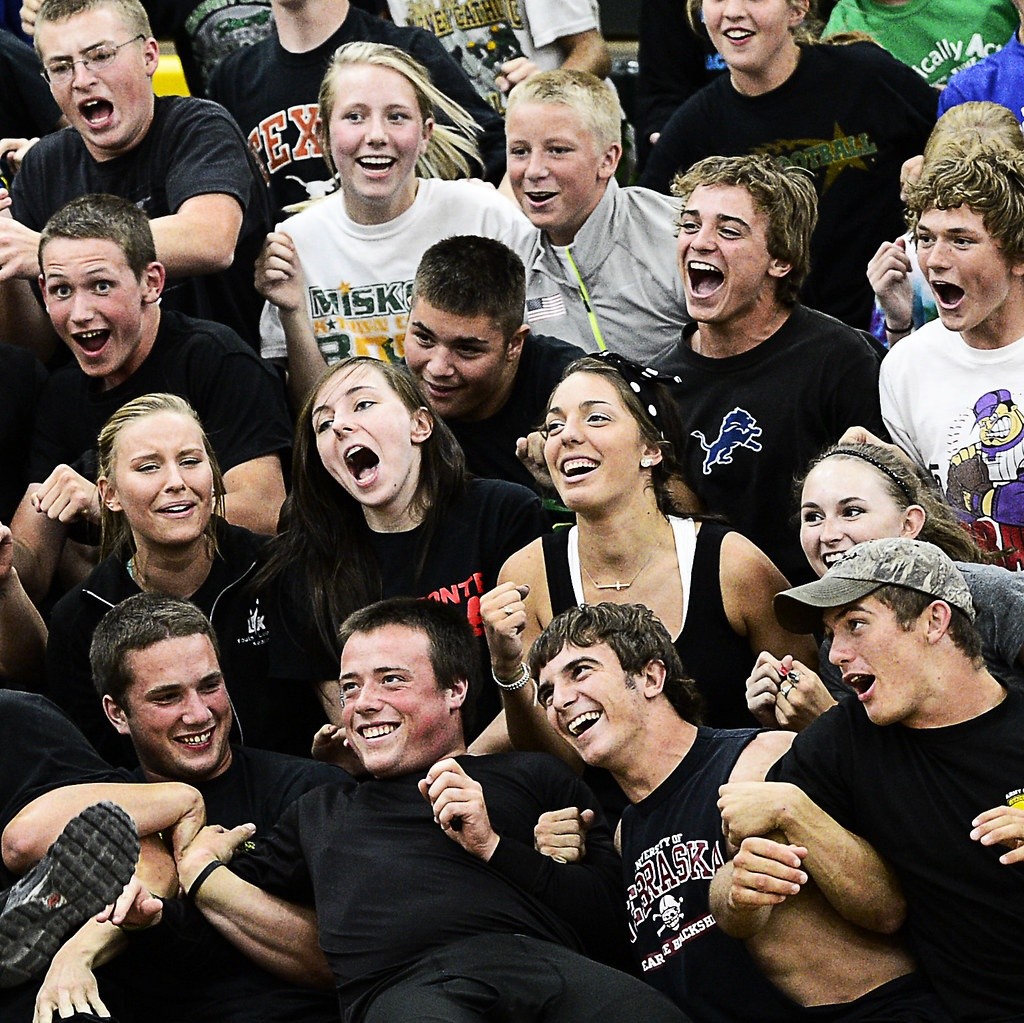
[884,319,914,333]
[188,860,224,898]
[491,662,538,707]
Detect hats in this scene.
[772,537,976,638]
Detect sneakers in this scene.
[0,800,140,991]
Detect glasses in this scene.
[40,34,146,86]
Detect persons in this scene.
[745,444,1024,733]
[177,598,688,1023]
[0,588,352,1023]
[480,351,817,835]
[44,392,277,773]
[533,602,957,1023]
[0,0,1024,621]
[267,357,551,785]
[0,524,179,1023]
[708,538,1024,1022]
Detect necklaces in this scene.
[580,543,660,591]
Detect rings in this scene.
[787,672,801,684]
[504,606,513,616]
[781,685,796,699]
